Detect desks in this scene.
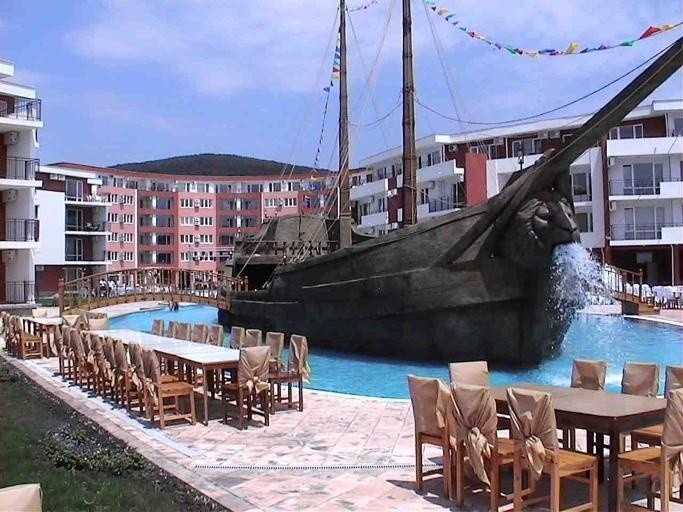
[490,384,668,512]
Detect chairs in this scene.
[568,358,683,499]
[267,334,308,415]
[505,387,598,512]
[54,319,284,427]
[616,388,680,512]
[449,361,488,387]
[409,375,469,499]
[0,307,108,362]
[447,382,531,512]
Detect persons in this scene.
[91,278,120,298]
[168,299,179,311]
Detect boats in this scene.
[214,0,683,374]
